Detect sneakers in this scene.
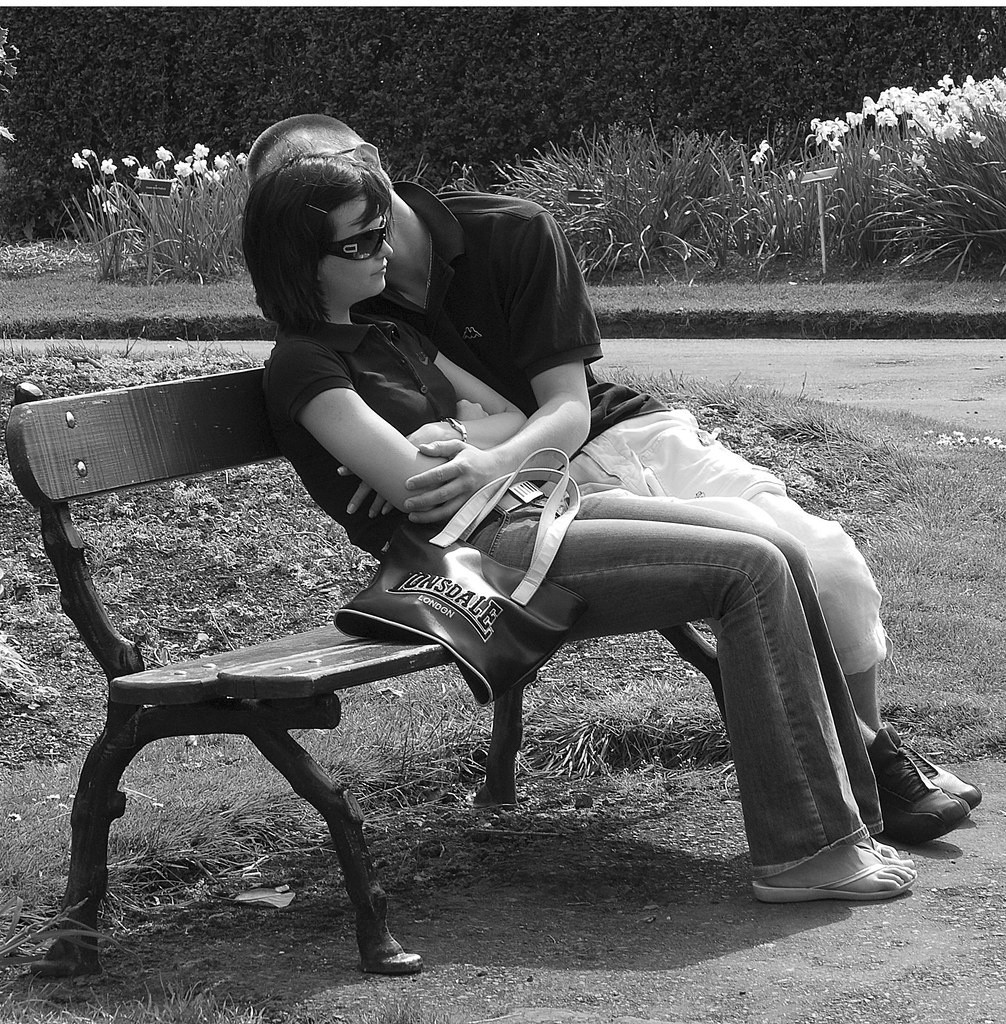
[880,720,982,810]
[867,729,971,845]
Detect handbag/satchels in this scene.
[335,448,590,708]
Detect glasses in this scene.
[326,216,387,261]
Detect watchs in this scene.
[446,417,468,441]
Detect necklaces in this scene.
[423,233,433,310]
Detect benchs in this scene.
[5,367,731,977]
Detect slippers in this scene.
[752,849,917,903]
[855,836,883,855]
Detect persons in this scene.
[245,110,984,846]
[240,148,918,903]
